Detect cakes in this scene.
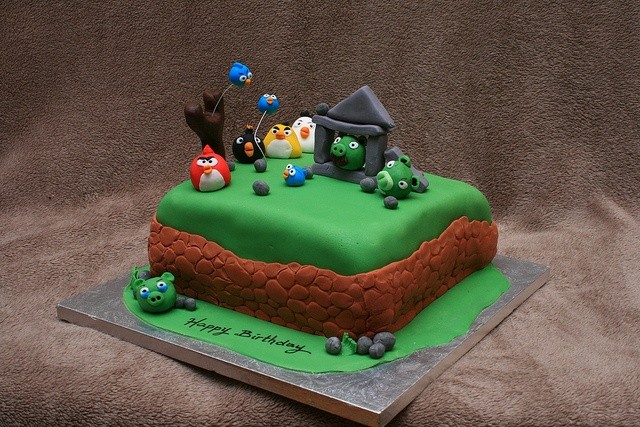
[123,62,509,374]
